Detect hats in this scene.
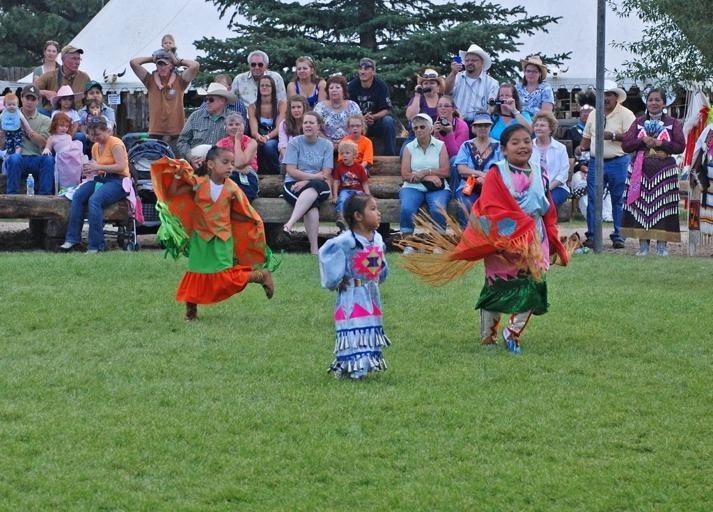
[156,58,169,65]
[413,113,433,124]
[197,82,237,103]
[359,58,376,67]
[61,46,83,56]
[521,56,547,80]
[459,44,491,71]
[592,80,627,103]
[471,114,494,126]
[22,80,102,104]
[417,68,446,93]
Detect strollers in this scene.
[120,137,175,253]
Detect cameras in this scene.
[417,86,432,94]
[439,117,450,127]
[489,98,503,106]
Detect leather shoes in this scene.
[613,240,625,248]
[583,239,593,247]
[56,245,75,252]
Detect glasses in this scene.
[413,126,425,129]
[251,63,263,67]
[422,74,436,78]
[204,97,214,102]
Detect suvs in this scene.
[549,81,594,119]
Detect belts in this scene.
[590,156,620,161]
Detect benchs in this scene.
[557,157,575,223]
[0,192,131,252]
[254,156,402,223]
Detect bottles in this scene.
[26,173,36,196]
[574,247,592,254]
[462,174,477,195]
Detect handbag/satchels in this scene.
[422,178,445,190]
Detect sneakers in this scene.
[501,330,520,351]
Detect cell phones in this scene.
[83,163,91,169]
[453,56,462,65]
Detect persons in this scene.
[0,33,713,256]
[392,124,580,354]
[150,146,281,322]
[318,193,392,381]
